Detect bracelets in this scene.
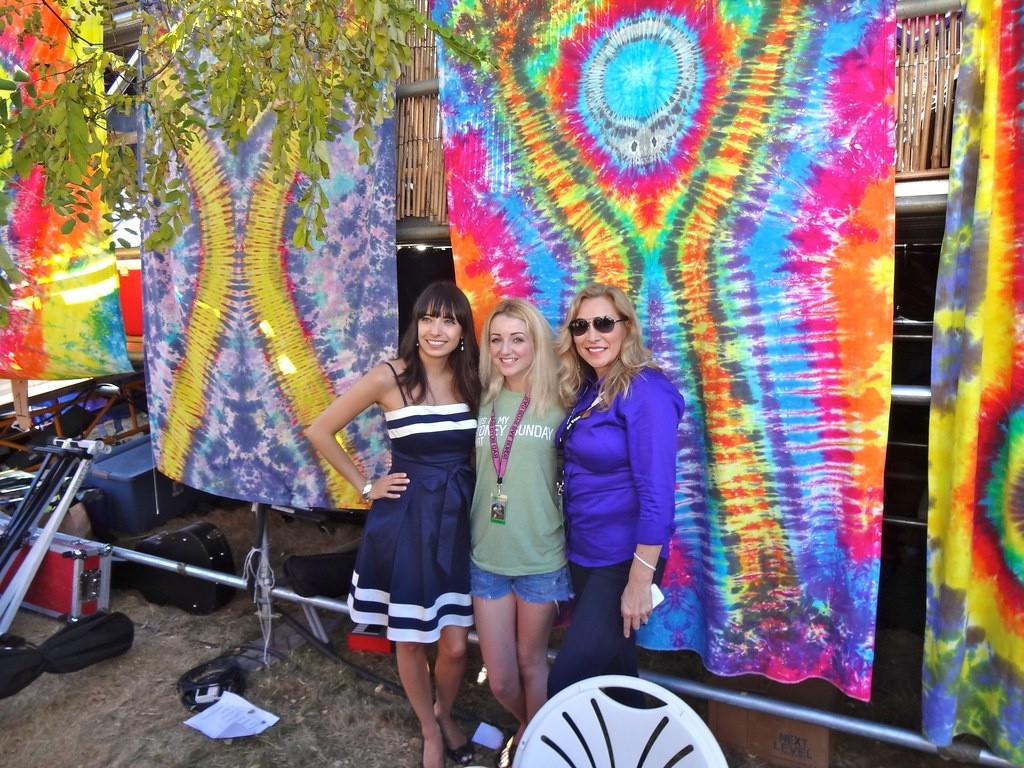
[633,553,656,571]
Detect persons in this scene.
[547,282,685,767]
[308,282,483,768]
[385,298,574,767]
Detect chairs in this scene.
[511,674,729,768]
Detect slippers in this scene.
[445,738,473,766]
[498,735,517,768]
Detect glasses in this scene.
[567,316,628,336]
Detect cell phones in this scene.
[651,583,665,609]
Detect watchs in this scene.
[362,480,375,502]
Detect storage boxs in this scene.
[708,672,837,768]
[81,433,193,534]
[0,519,113,625]
[347,623,392,654]
